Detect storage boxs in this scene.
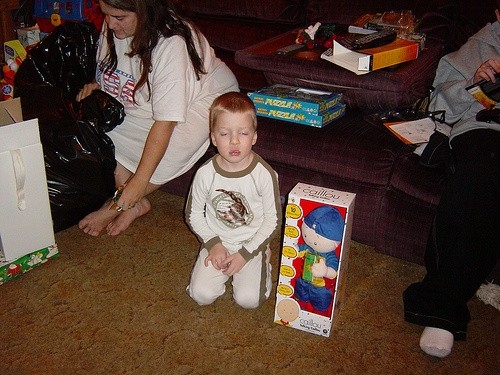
[326,36,420,76]
[273,183,355,339]
[0,98,60,285]
[246,83,345,128]
[4,0,90,70]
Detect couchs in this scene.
[169,0,499,285]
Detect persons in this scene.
[185,91,282,308]
[402,0,500,357]
[76,0,241,237]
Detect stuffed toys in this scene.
[294,205,344,312]
[0,59,17,101]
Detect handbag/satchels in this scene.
[75,90,125,132]
[38,120,116,234]
[13,21,101,119]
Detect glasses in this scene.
[400,106,446,120]
